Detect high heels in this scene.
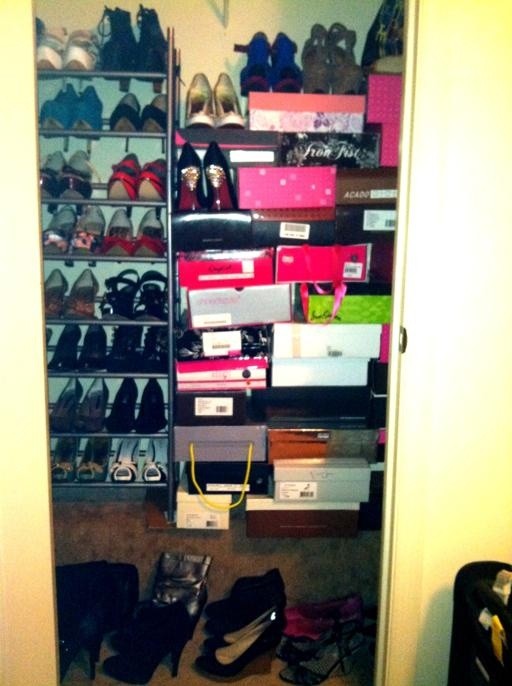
[73,377,109,433]
[184,73,215,128]
[135,378,167,433]
[44,269,68,320]
[104,376,138,435]
[213,73,246,130]
[68,85,104,131]
[203,141,237,211]
[42,205,77,256]
[58,608,100,682]
[301,24,330,94]
[77,324,107,372]
[233,31,271,97]
[39,150,66,200]
[51,437,80,482]
[327,22,364,95]
[132,270,167,322]
[173,143,206,209]
[41,81,78,132]
[140,94,167,132]
[75,437,112,483]
[135,6,166,73]
[137,158,166,202]
[107,153,140,201]
[141,438,168,482]
[101,584,208,685]
[37,34,62,71]
[269,32,302,92]
[134,327,168,373]
[63,268,99,320]
[133,209,165,257]
[105,324,143,373]
[98,268,140,321]
[96,5,137,72]
[277,591,367,686]
[49,377,83,434]
[62,30,95,72]
[109,92,141,133]
[47,324,82,372]
[192,567,286,681]
[58,151,93,200]
[109,438,140,482]
[68,206,106,256]
[102,209,135,257]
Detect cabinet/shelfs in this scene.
[168,26,406,532]
[34,26,169,518]
